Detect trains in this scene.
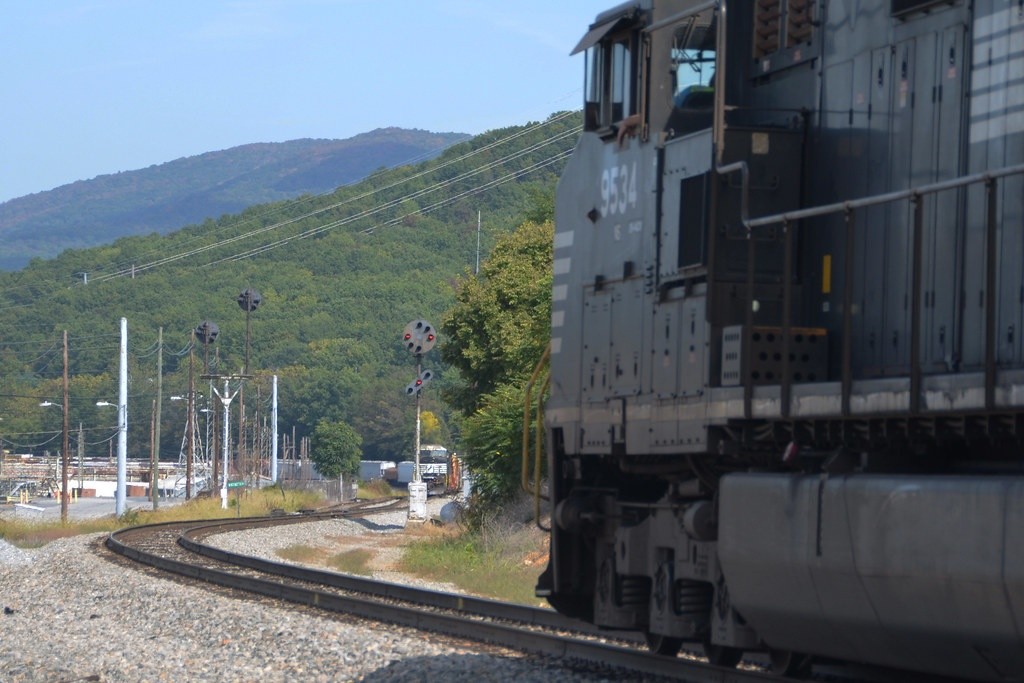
[521,0,1024,683]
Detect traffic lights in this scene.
[405,367,433,398]
[401,319,437,356]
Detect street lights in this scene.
[168,388,215,497]
[39,329,72,523]
[94,317,127,519]
